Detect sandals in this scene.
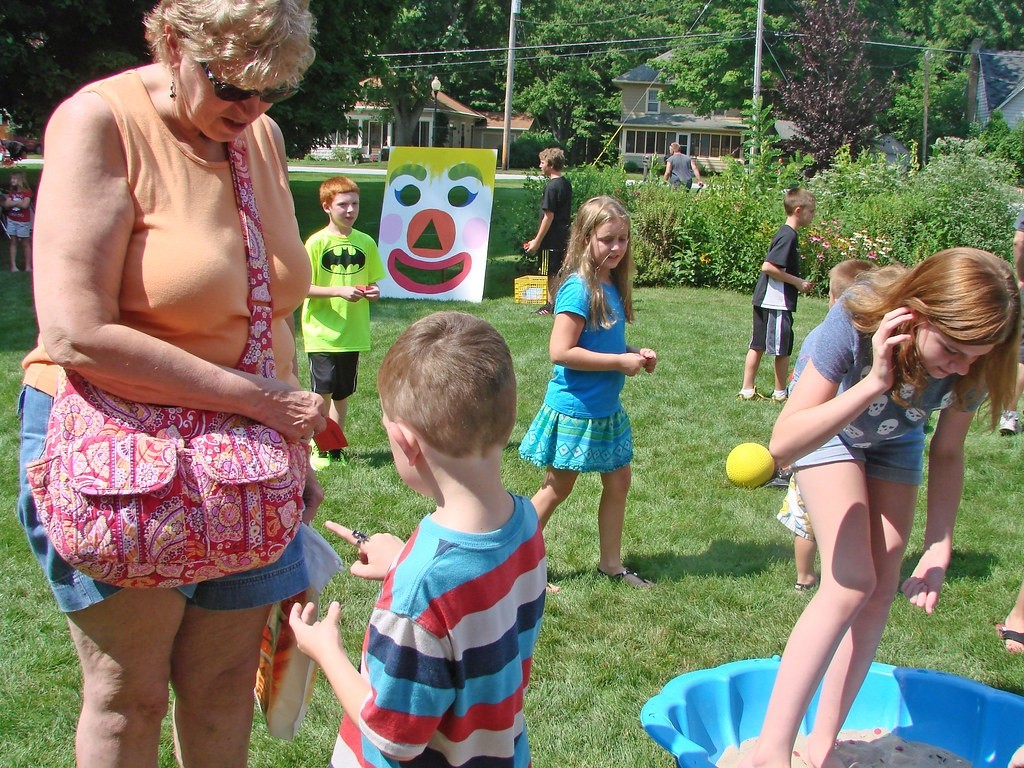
[597,564,649,587]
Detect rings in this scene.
[352,530,369,547]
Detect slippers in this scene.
[996,623,1024,655]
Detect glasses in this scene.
[200,61,299,103]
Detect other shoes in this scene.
[794,583,817,590]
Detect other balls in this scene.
[725,443,774,488]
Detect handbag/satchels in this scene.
[27,356,310,588]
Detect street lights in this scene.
[431,75,441,147]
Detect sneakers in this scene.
[308,437,332,472]
[736,386,771,403]
[770,389,788,405]
[531,301,556,315]
[999,411,1019,436]
[329,448,347,468]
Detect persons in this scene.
[21,0,347,768]
[773,255,883,591]
[997,208,1023,435]
[748,243,1023,768]
[736,184,815,403]
[289,307,548,768]
[663,141,704,194]
[525,147,572,318]
[1,107,15,163]
[518,191,658,588]
[4,170,36,272]
[296,176,383,473]
